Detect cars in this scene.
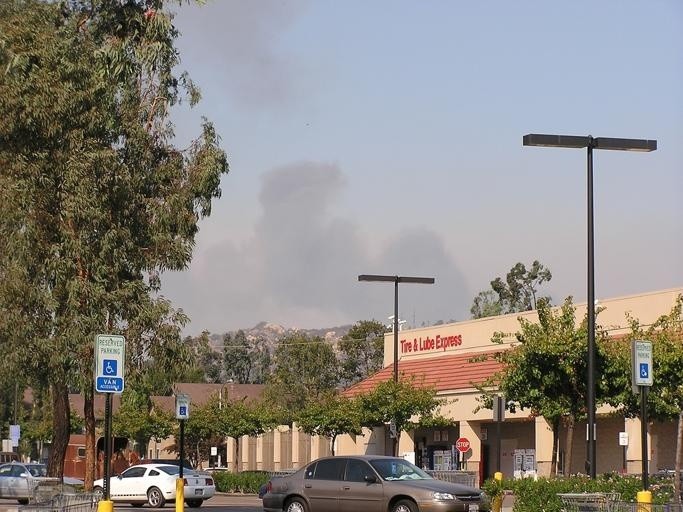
[261,456,490,512]
[93,463,215,509]
[0,462,84,506]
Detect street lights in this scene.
[217,379,233,468]
[357,273,434,462]
[522,130,657,482]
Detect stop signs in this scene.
[455,438,470,453]
